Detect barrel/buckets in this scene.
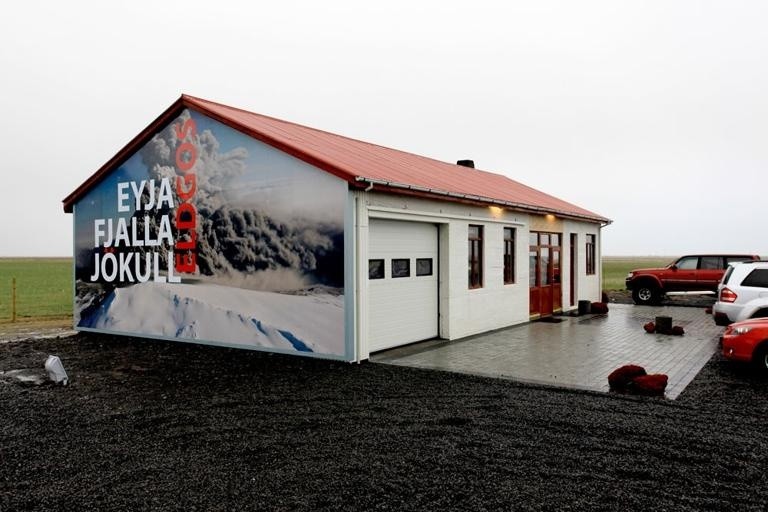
[656,316,672,335]
[579,300,591,314]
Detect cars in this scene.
[717,317,768,374]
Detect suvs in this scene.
[625,254,761,305]
[712,260,768,326]
